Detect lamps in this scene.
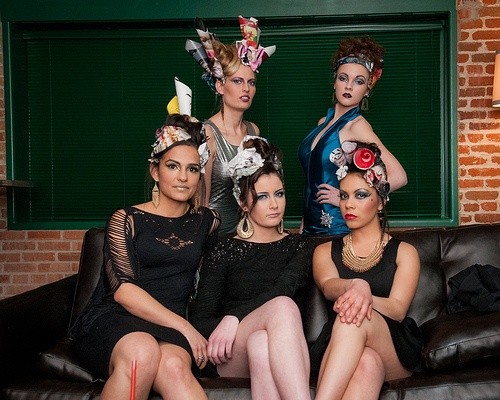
[492,50,500,108]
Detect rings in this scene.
[199,354,205,362]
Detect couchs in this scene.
[0,223,500,400]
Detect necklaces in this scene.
[341,229,386,272]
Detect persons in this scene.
[298,37,408,237]
[74,114,222,400]
[194,135,314,400]
[185,14,277,235]
[312,138,420,400]
